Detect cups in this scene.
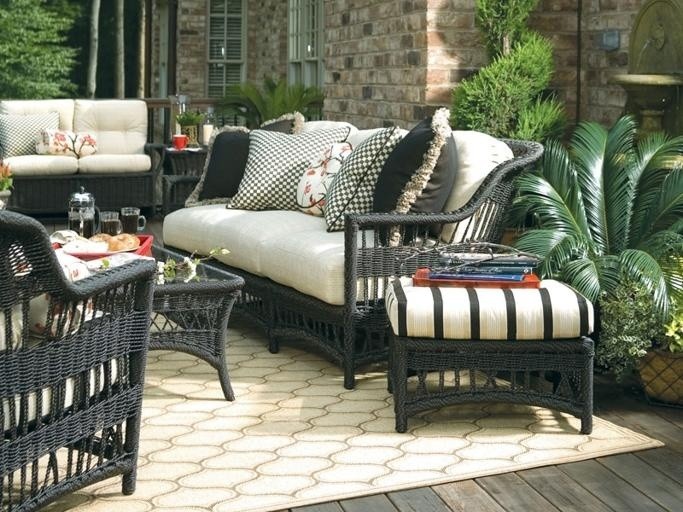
[97,209,123,236]
[171,133,188,150]
[120,207,146,237]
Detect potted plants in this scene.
[173,108,205,145]
[629,254,682,410]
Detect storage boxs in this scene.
[47,234,153,262]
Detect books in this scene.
[412,253,541,288]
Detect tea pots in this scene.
[166,94,190,143]
[66,184,99,238]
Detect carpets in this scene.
[0,274,665,511]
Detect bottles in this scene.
[201,113,213,148]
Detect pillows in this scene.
[321,119,401,234]
[228,127,350,209]
[0,111,64,159]
[31,129,98,155]
[183,110,303,210]
[14,251,92,342]
[295,140,359,217]
[370,107,457,255]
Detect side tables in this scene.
[154,143,210,225]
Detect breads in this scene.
[109,233,140,251]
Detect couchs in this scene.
[378,270,598,433]
[0,89,167,229]
[157,103,545,393]
[0,205,159,511]
